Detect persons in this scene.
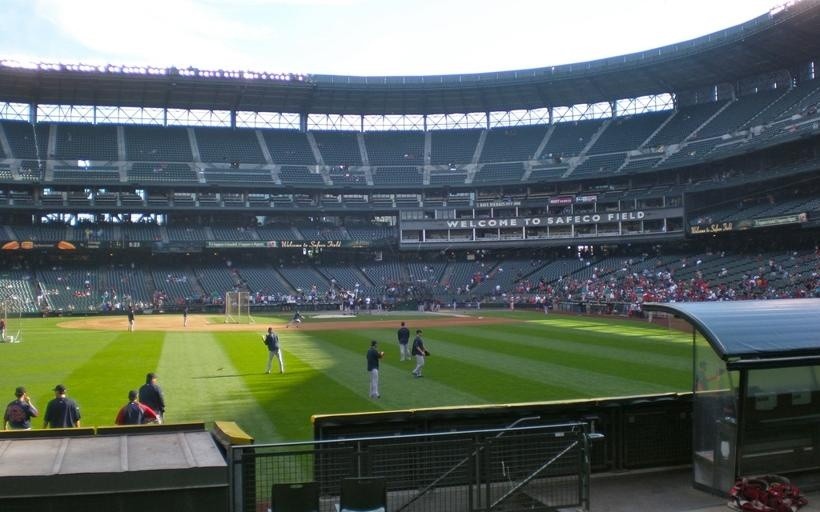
[286,309,305,328]
[42,383,82,428]
[0,225,820,320]
[114,390,158,425]
[127,308,134,331]
[137,371,166,424]
[396,321,412,361]
[410,329,431,378]
[0,318,6,342]
[183,306,187,326]
[2,386,40,430]
[365,340,385,399]
[261,327,284,374]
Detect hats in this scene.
[14,387,26,396]
[51,384,66,393]
[146,373,159,380]
[128,391,138,400]
